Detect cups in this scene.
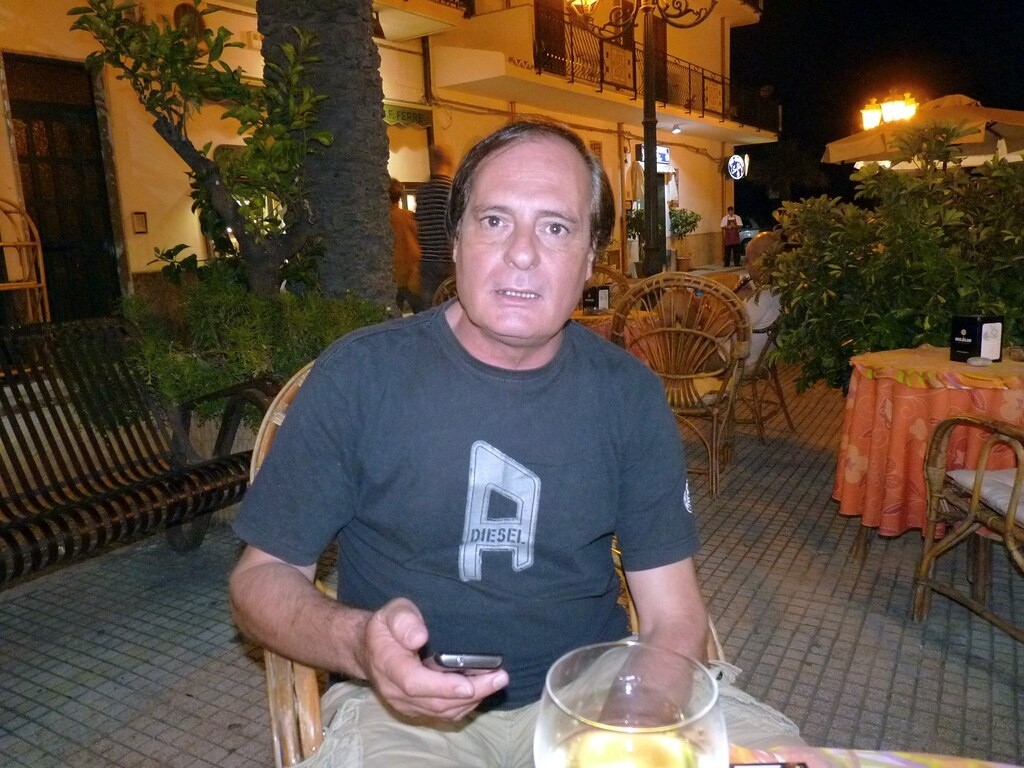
[532,640,729,768]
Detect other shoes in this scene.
[723,264,730,267]
[734,264,742,267]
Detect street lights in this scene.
[568,0,713,313]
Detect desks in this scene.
[831,347,1024,563]
[569,306,661,367]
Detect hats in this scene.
[389,179,405,194]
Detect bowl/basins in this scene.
[1009,345,1024,362]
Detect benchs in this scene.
[0,320,282,585]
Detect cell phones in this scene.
[420,652,506,676]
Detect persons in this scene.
[715,232,781,365]
[228,122,808,768]
[390,178,420,311]
[722,207,743,267]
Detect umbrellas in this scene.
[820,93,1024,171]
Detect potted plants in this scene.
[669,209,700,273]
[625,208,649,279]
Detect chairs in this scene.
[431,263,797,500]
[905,415,1024,644]
[247,356,724,768]
